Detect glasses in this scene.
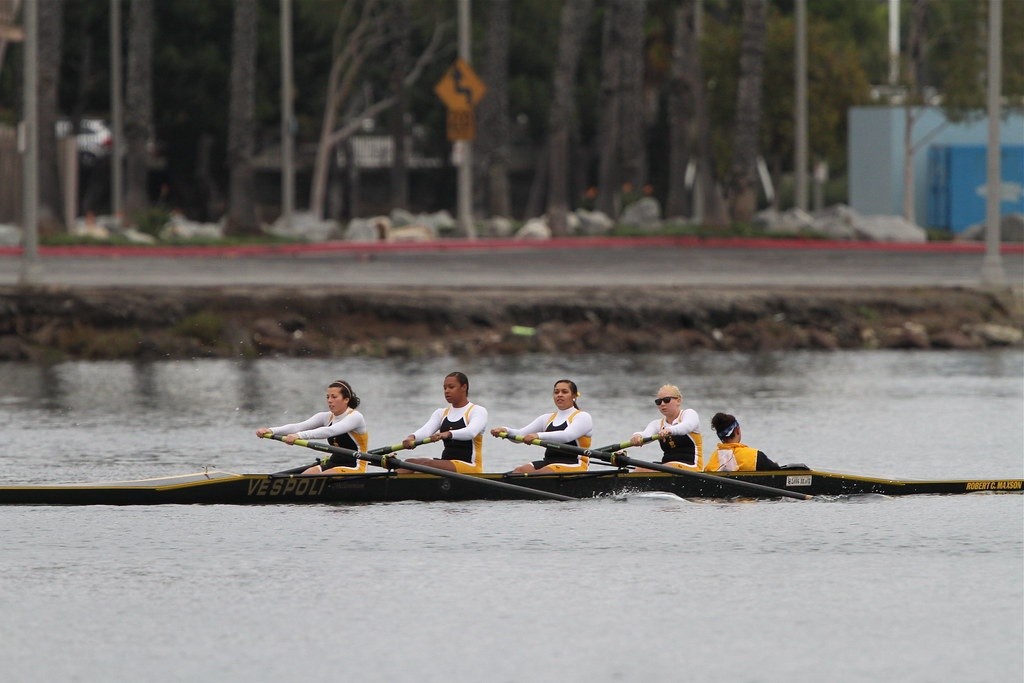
[654,397,678,405]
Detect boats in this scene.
[0,466,1019,505]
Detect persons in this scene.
[256,380,369,474]
[492,379,592,474]
[632,385,703,472]
[704,412,814,472]
[398,371,489,474]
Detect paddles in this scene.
[497,430,820,500]
[589,434,668,456]
[276,438,437,474]
[260,433,576,503]
